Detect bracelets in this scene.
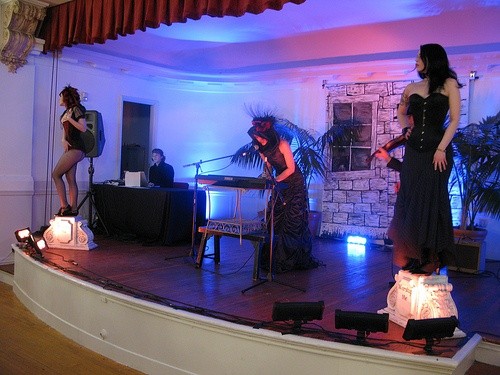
[437,149,446,152]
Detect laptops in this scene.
[126,171,149,187]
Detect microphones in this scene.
[243,145,259,157]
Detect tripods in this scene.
[240,152,308,293]
[78,159,109,236]
[163,152,237,268]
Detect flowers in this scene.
[251,121,271,132]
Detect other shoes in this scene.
[68,209,78,216]
[54,204,71,216]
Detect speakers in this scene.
[81,110,106,157]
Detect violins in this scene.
[365,134,405,165]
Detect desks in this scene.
[92,182,207,247]
[196,226,266,281]
[203,185,270,246]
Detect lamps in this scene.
[15,227,33,246]
[270,300,325,331]
[34,237,49,259]
[402,315,459,352]
[335,309,389,342]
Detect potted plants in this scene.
[448,113,500,274]
[230,103,363,238]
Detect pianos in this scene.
[164,174,307,294]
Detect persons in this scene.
[144,148,174,186]
[247,114,322,279]
[52,83,87,217]
[376,44,463,275]
